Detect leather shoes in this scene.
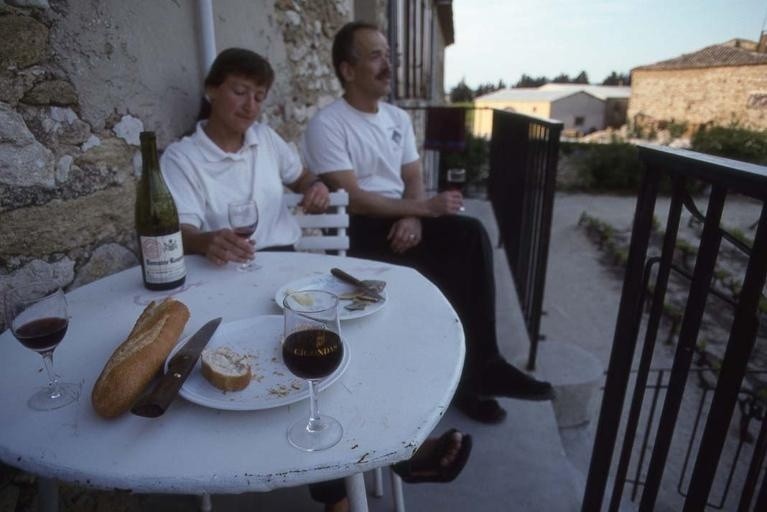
[452,393,507,424]
[468,360,557,400]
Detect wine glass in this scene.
[227,199,263,275]
[4,279,82,414]
[279,288,345,453]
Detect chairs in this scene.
[198,188,406,512]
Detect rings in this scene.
[410,231,417,240]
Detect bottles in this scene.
[134,130,188,293]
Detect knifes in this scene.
[330,267,386,302]
[129,316,223,420]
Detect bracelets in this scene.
[311,179,321,187]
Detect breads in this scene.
[91,297,190,418]
[200,346,252,391]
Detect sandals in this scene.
[394,429,469,484]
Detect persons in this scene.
[298,20,556,425]
[149,47,473,511]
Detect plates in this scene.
[163,313,352,414]
[274,272,390,323]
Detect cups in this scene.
[445,168,467,213]
